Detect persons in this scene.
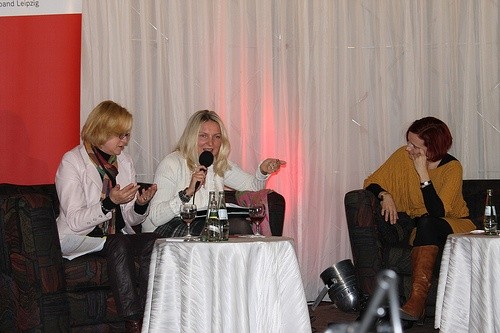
[55,101,161,333]
[141,110,286,239]
[363,116,477,325]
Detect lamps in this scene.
[311,259,370,312]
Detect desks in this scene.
[140,230,500,333]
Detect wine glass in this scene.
[249,203,266,239]
[179,203,197,239]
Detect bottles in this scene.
[207,200,221,242]
[484,189,498,235]
[200,191,215,239]
[216,192,229,241]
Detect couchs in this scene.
[0,182,285,333]
[344,179,500,322]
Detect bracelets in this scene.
[420,180,431,185]
[100,204,111,211]
[179,187,193,203]
[381,192,390,200]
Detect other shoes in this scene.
[123,313,144,333]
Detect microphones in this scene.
[195,151,214,191]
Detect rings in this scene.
[144,197,147,200]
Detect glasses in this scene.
[116,131,130,140]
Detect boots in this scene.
[401,244,438,319]
